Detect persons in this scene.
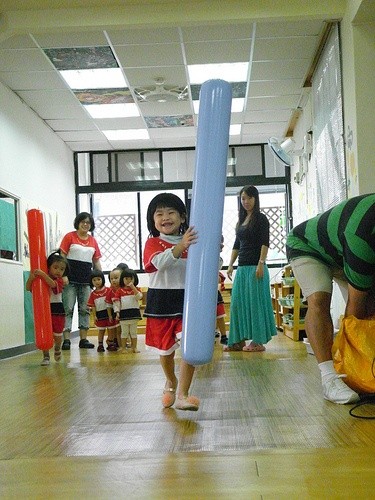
[26,249,69,365]
[286,193,375,404]
[144,193,223,411]
[87,263,229,353]
[60,212,102,350]
[224,185,277,352]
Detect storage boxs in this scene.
[88,283,233,327]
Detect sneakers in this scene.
[321,373,360,403]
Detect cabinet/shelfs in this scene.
[271,267,308,340]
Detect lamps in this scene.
[134,85,189,104]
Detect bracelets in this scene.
[259,260,264,264]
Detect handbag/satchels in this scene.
[333,315,375,394]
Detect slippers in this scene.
[223,340,246,351]
[242,341,265,351]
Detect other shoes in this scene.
[119,348,128,354]
[79,339,94,349]
[126,343,131,348]
[114,338,119,348]
[175,394,199,411]
[54,346,61,361]
[62,339,70,350]
[130,347,141,353]
[215,331,220,337]
[106,339,116,351]
[98,346,105,352]
[161,379,178,408]
[41,355,50,365]
[220,337,228,344]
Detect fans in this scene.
[268,136,299,167]
